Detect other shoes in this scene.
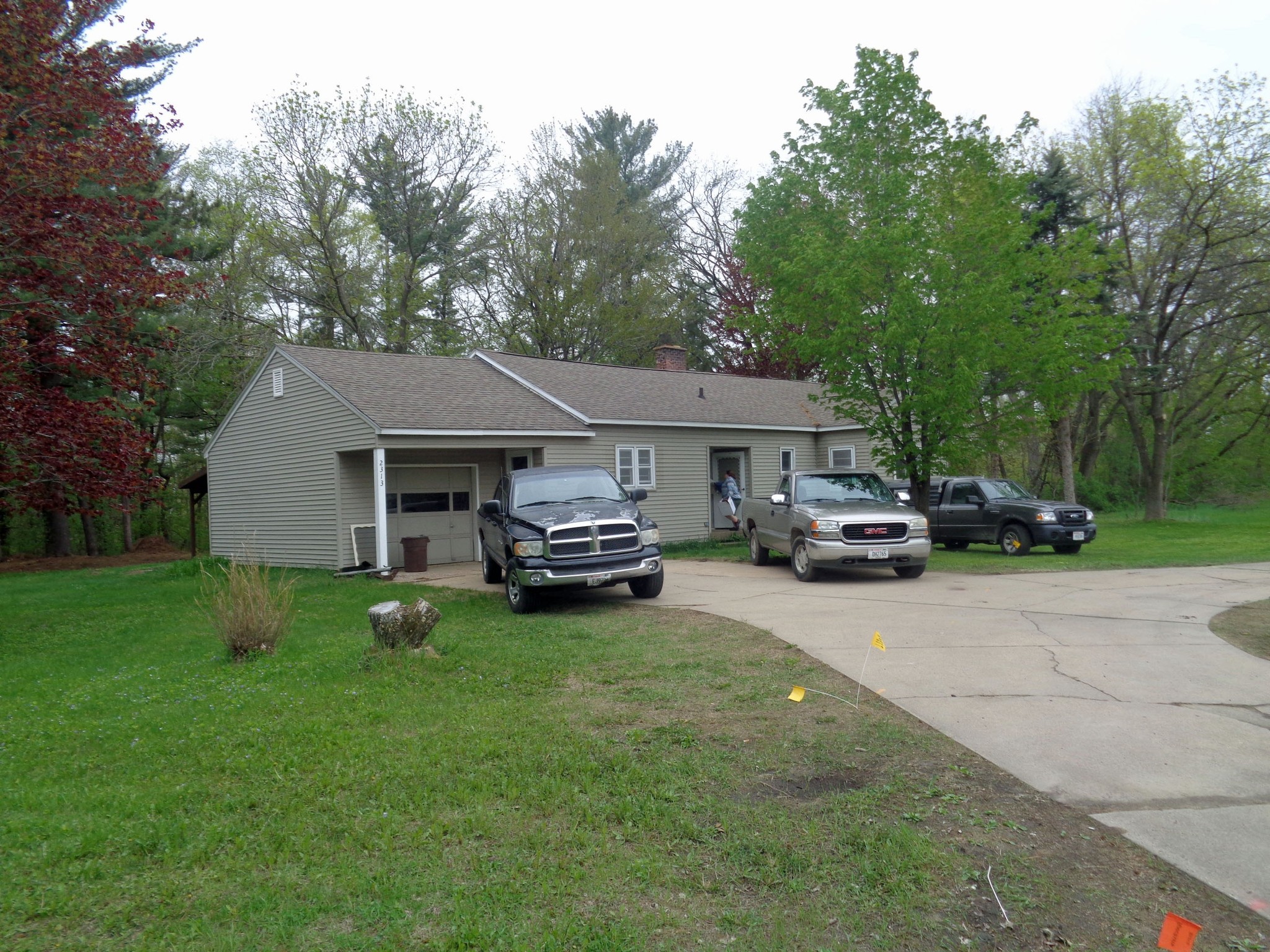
[729,527,738,531]
[734,520,741,529]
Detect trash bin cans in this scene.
[400,537,430,573]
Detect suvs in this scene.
[477,464,664,615]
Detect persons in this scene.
[721,470,741,531]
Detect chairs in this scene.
[798,486,807,499]
[952,488,965,499]
[841,483,858,496]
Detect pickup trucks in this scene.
[885,476,1097,556]
[742,469,931,582]
